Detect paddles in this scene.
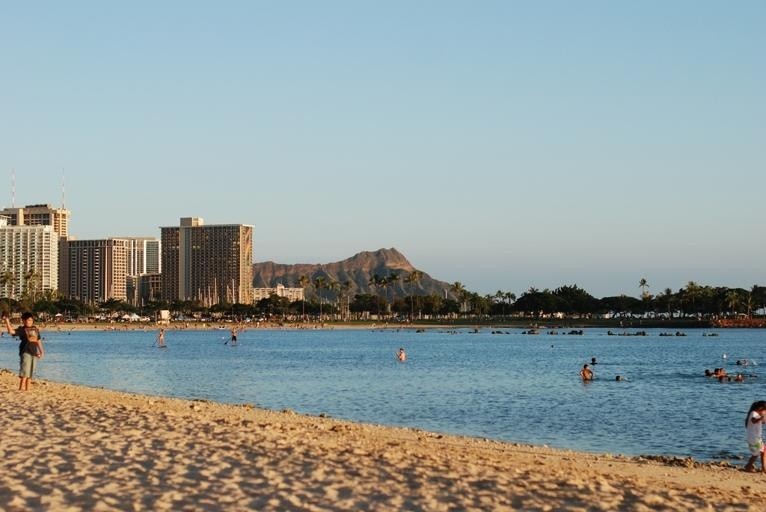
[224,328,240,344]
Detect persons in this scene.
[615,374,624,381]
[231,326,238,344]
[704,358,751,384]
[590,357,599,367]
[159,328,166,347]
[396,346,406,363]
[744,400,766,474]
[578,362,595,381]
[3,312,45,392]
